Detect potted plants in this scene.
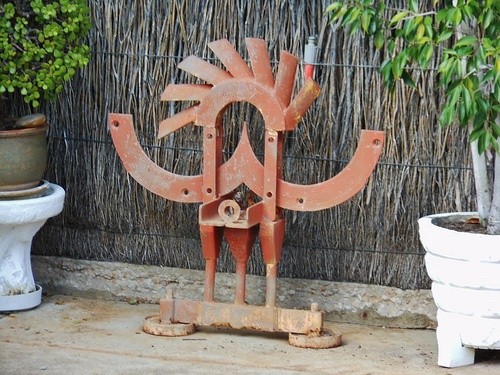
[328,0,500,349]
[0,0,92,193]
